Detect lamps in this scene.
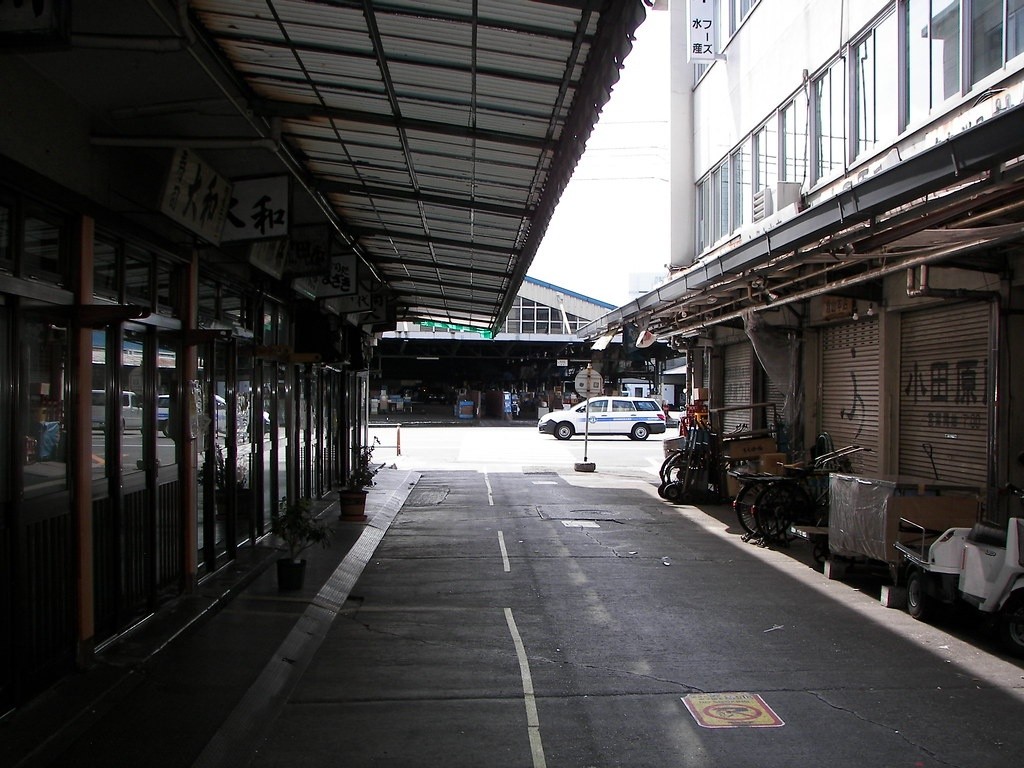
[634,330,656,349]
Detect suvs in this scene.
[537,395,667,441]
[155,393,274,440]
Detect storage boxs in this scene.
[371,390,411,415]
[828,473,979,564]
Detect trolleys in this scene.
[791,524,832,565]
[657,414,712,505]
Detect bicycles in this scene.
[726,443,873,548]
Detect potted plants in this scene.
[337,444,378,523]
[197,441,247,520]
[270,495,338,592]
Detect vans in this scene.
[88,389,147,433]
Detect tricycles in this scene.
[892,481,1024,661]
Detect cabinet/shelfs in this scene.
[709,401,780,502]
